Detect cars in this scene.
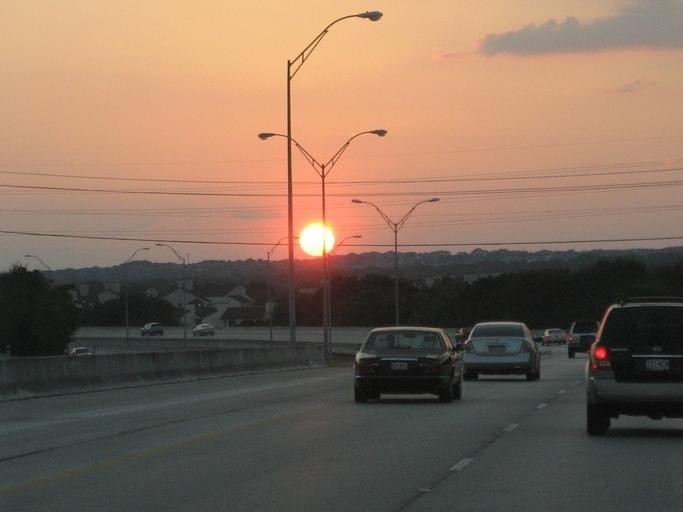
[192,324,216,336]
[532,297,683,435]
[353,321,541,403]
[70,347,93,356]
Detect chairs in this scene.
[376,335,437,349]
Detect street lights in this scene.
[125,244,186,347]
[258,129,388,364]
[286,9,385,339]
[25,255,50,272]
[352,198,442,344]
[328,235,364,346]
[266,236,302,347]
